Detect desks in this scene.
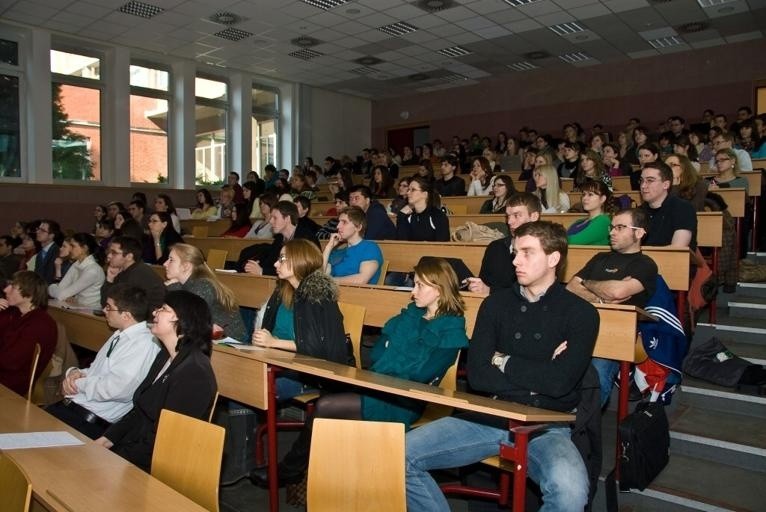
[0,156,766,511]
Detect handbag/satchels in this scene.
[682,336,765,388]
[452,221,505,243]
[737,259,766,282]
[618,403,670,492]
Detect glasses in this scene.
[35,227,49,234]
[106,304,126,311]
[278,256,288,263]
[608,224,646,232]
[715,158,731,164]
[492,184,506,187]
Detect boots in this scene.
[221,413,311,487]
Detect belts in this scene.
[61,398,111,430]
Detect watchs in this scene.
[492,352,509,369]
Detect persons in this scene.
[564,210,659,413]
[43,283,163,439]
[220,238,345,488]
[404,220,600,512]
[1,107,764,344]
[1,271,58,400]
[93,290,218,478]
[249,255,471,489]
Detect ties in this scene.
[40,250,46,260]
